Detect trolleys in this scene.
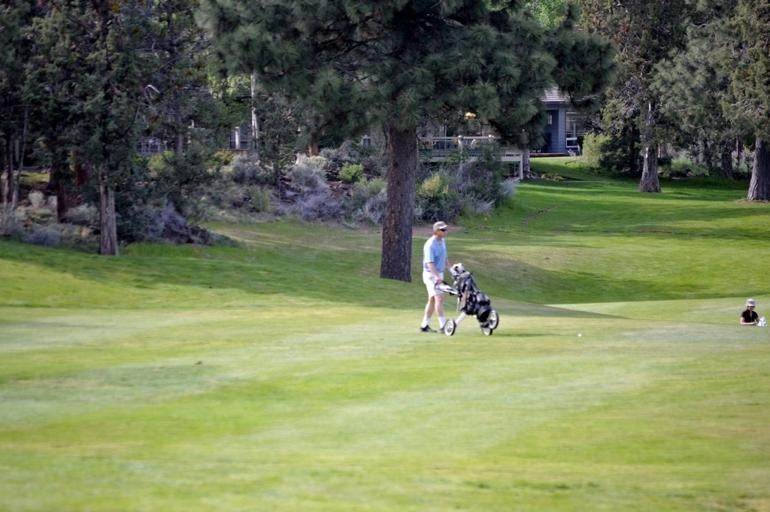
[433,264,499,337]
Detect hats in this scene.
[433,221,448,233]
[746,300,755,306]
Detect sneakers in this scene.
[419,326,437,333]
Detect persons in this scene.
[740,299,760,326]
[417,220,449,333]
